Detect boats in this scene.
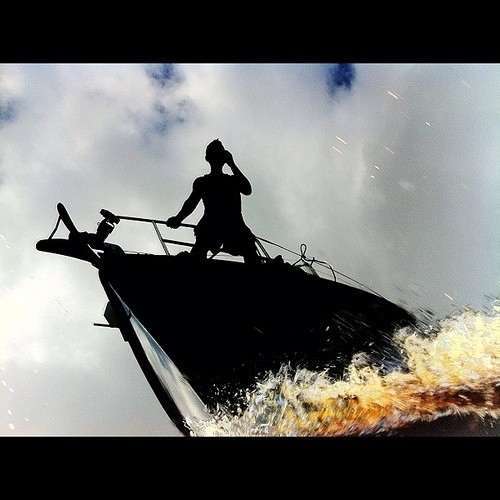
[32,200,418,438]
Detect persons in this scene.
[167,139,262,265]
